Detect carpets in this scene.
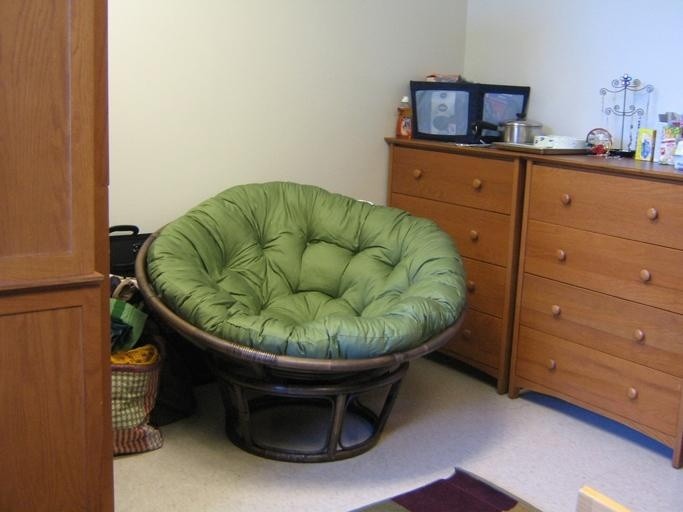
[348,466,545,511]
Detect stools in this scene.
[135,181,464,463]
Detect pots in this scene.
[481,113,543,144]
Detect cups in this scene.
[672,153,683,170]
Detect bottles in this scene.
[395,95,414,141]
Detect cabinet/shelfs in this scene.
[0,2,113,511]
[508,153,683,470]
[386,137,525,394]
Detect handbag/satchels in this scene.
[110,232,195,456]
